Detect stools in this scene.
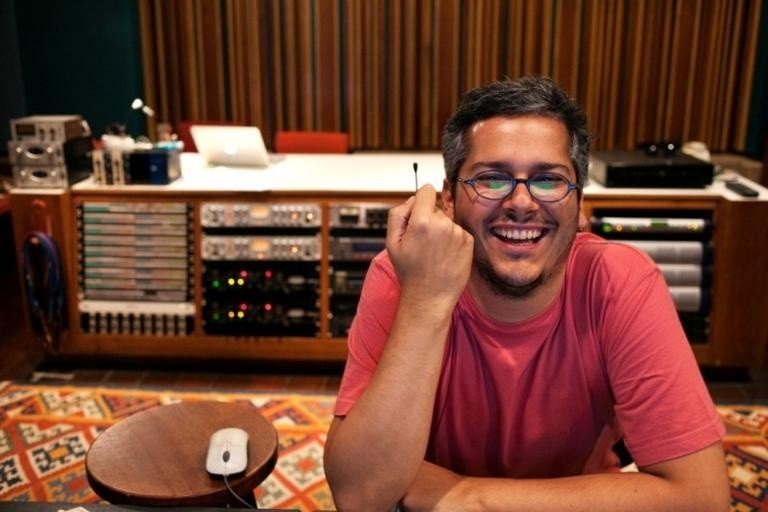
[85,401,279,505]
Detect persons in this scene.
[323,74,731,511]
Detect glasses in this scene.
[456,171,579,203]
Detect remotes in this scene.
[727,180,759,197]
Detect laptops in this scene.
[189,125,287,166]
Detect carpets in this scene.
[0,380,767,512]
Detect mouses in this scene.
[204,427,250,480]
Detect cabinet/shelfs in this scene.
[10,150,768,383]
